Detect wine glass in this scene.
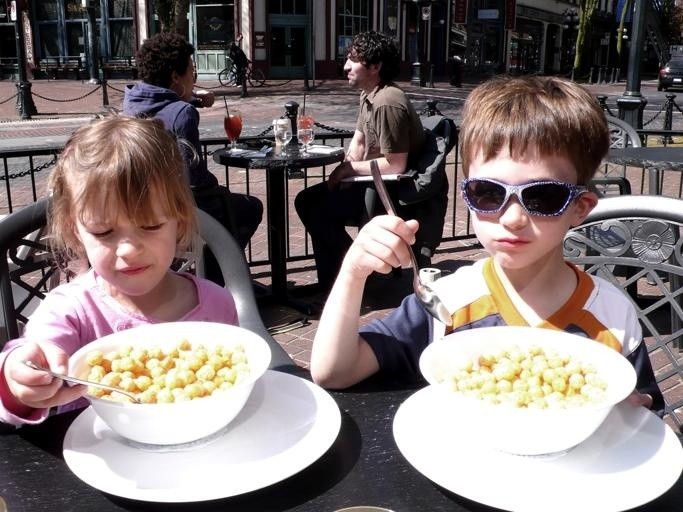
[272,119,293,156]
[297,124,315,158]
[224,112,242,155]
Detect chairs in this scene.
[591,114,640,299]
[566,193,683,438]
[343,114,457,306]
[0,197,299,366]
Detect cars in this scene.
[657,59,682,91]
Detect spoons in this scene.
[370,160,453,328]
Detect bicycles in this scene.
[219,54,266,87]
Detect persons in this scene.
[230,46,244,88]
[309,74,665,420]
[121,33,264,289]
[0,113,240,427]
[294,30,426,291]
[227,33,242,82]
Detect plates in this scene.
[393,386,682,511]
[63,368,342,504]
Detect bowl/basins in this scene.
[65,322,272,444]
[417,326,637,454]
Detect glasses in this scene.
[461,177,589,218]
